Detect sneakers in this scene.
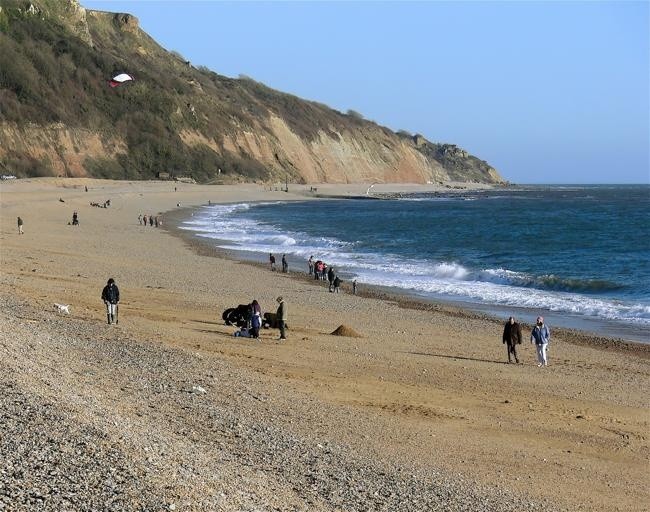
[280,336,286,340]
[507,359,519,363]
[538,361,547,367]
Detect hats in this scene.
[537,316,543,321]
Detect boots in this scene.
[107,313,115,324]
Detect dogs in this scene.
[53,302,71,315]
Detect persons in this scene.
[276,296,287,340]
[530,317,550,367]
[503,316,523,363]
[248,300,261,328]
[60,185,159,228]
[17,216,24,235]
[101,278,119,325]
[250,313,260,338]
[270,253,358,294]
